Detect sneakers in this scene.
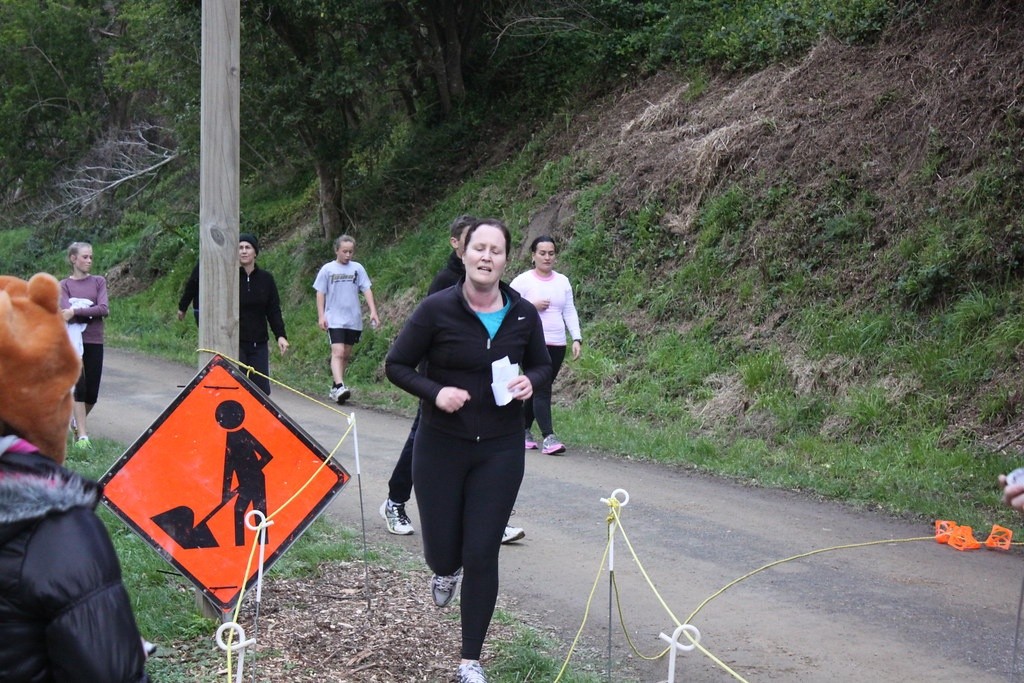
[524,428,539,449]
[431,565,462,606]
[378,498,414,534]
[75,439,95,454]
[335,386,350,405]
[542,434,566,455]
[70,419,79,446]
[329,386,337,398]
[456,660,486,683]
[499,509,525,544]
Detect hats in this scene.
[240,232,258,256]
[0,272,81,466]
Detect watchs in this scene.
[574,339,583,345]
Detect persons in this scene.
[386,220,553,683]
[379,220,526,544]
[0,271,159,683]
[312,235,381,404]
[57,237,111,447]
[178,256,199,328]
[997,468,1024,513]
[508,236,583,455]
[239,232,290,396]
